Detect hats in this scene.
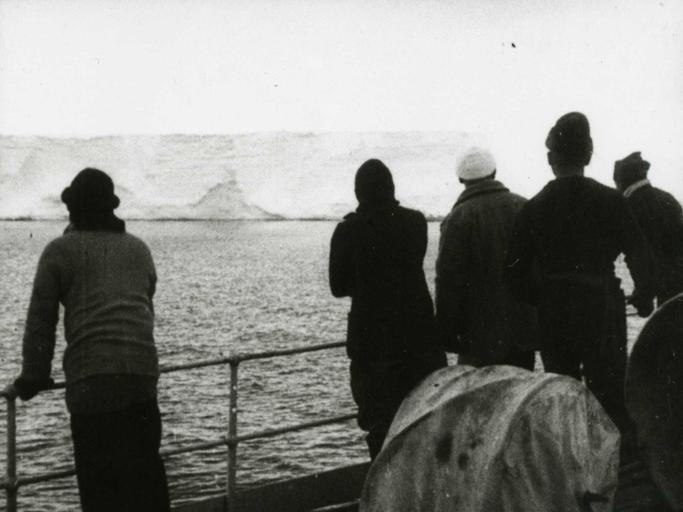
[355,159,395,203]
[61,167,120,212]
[546,112,594,160]
[457,146,497,182]
[613,152,650,183]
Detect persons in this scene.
[2,167,172,512]
[613,150,683,311]
[328,159,450,464]
[435,145,539,372]
[501,109,663,436]
